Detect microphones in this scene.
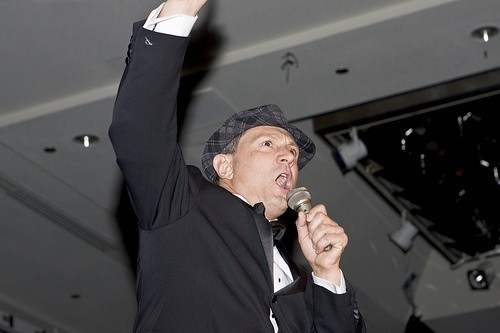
[286,186,333,250]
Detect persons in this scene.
[108,0,369,333]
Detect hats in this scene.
[202,105,316,184]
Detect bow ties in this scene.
[253,203,287,240]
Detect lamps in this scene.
[331,140,367,177]
[466,262,496,291]
[388,222,418,253]
